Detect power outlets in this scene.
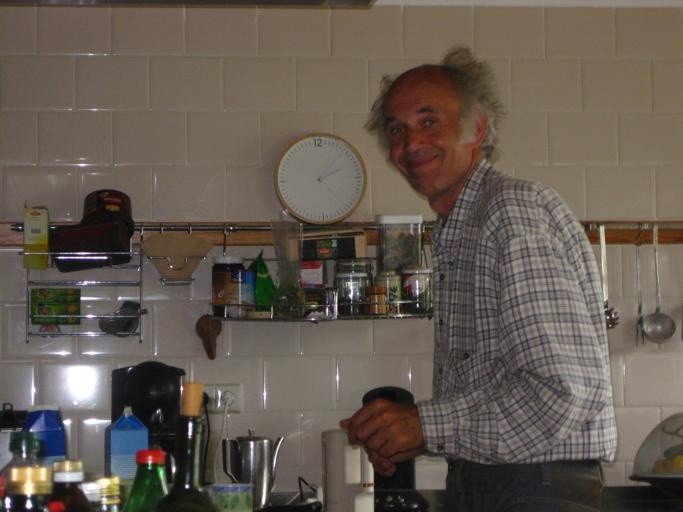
[198,383,243,414]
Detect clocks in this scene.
[271,131,370,229]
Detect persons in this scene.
[336,43,620,510]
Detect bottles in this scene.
[0,393,255,510]
[288,254,436,315]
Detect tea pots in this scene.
[221,428,286,511]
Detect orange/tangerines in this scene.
[653,454,682,473]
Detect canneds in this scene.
[400,268,434,314]
[333,258,374,315]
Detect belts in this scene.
[445,453,606,484]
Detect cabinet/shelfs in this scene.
[18,223,145,344]
